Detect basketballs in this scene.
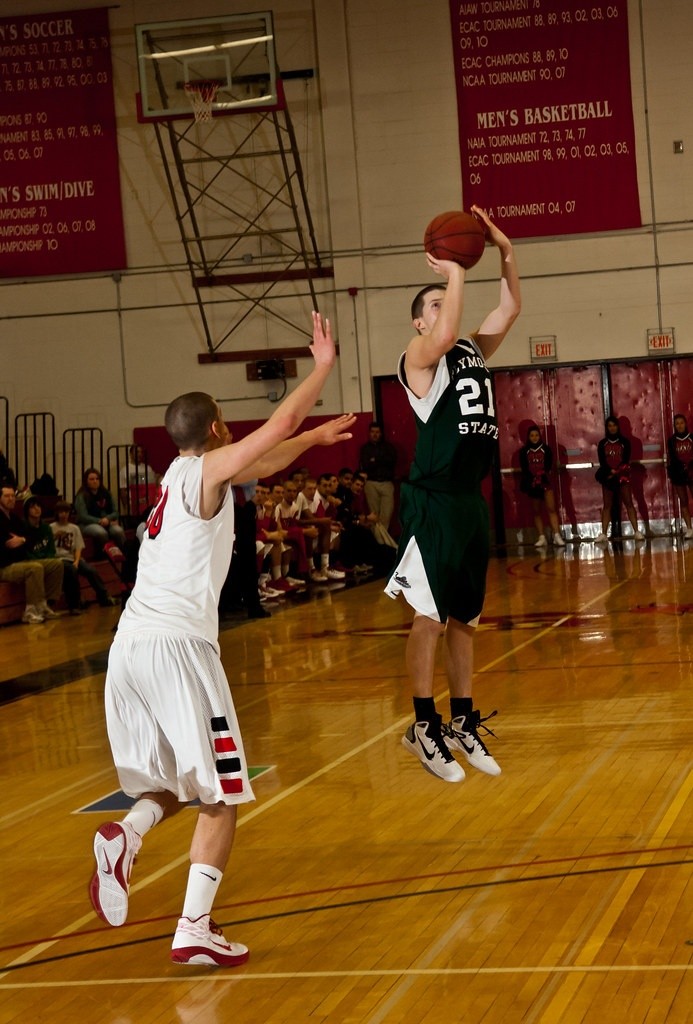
[423,210,486,271]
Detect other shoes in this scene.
[99,597,121,606]
[69,607,86,615]
[248,608,271,618]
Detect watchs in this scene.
[22,537,26,543]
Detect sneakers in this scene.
[685,529,693,538]
[36,606,59,618]
[594,542,609,550]
[552,533,565,545]
[401,714,465,783]
[593,534,608,542]
[260,574,368,607]
[37,620,62,637]
[88,821,142,927]
[170,914,250,967]
[635,540,644,548]
[535,547,548,554]
[173,962,251,1021]
[22,624,44,639]
[22,610,44,623]
[257,562,372,600]
[535,535,547,546]
[441,710,502,776]
[553,546,565,555]
[634,531,645,539]
[685,539,693,546]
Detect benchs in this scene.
[0,495,127,622]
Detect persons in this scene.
[359,422,398,532]
[382,205,523,783]
[0,468,165,625]
[519,426,565,547]
[669,414,693,538]
[215,424,270,620]
[88,312,356,968]
[118,444,157,516]
[594,416,645,542]
[251,466,372,602]
